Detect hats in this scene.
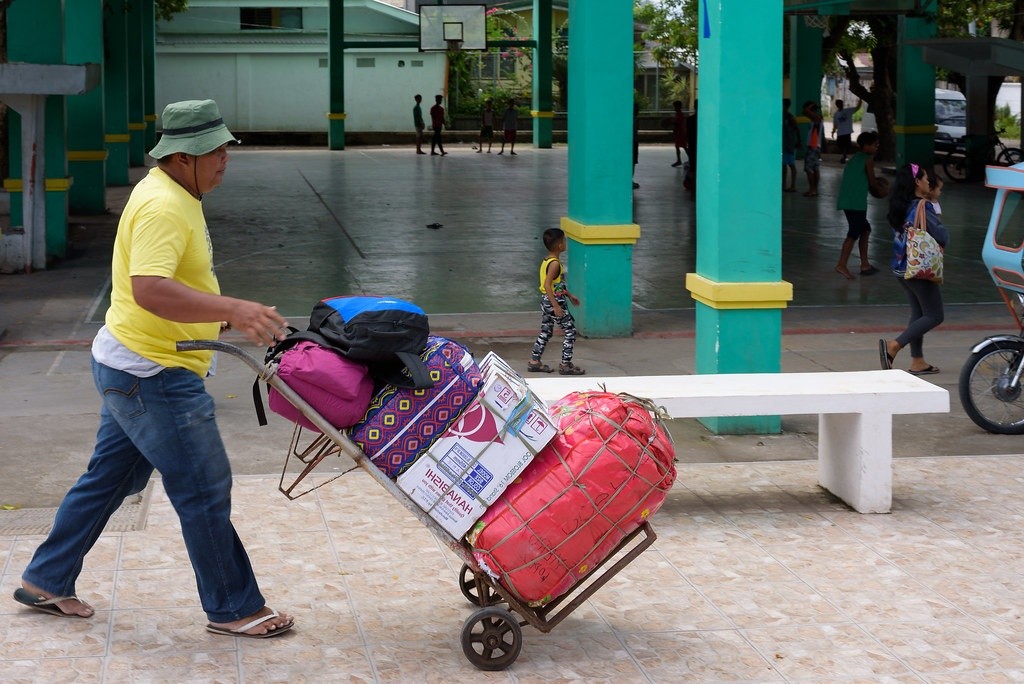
[149,100,242,160]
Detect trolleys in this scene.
[177,323,658,672]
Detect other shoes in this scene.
[416,150,517,156]
[632,182,641,189]
[672,160,682,166]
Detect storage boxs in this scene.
[395,351,556,542]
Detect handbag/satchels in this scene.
[904,199,945,283]
[821,137,829,155]
[347,335,484,483]
[268,342,373,432]
[310,296,433,389]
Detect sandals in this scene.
[558,361,586,375]
[528,361,554,373]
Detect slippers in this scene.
[14,587,95,619]
[835,265,854,280]
[804,192,819,197]
[909,364,940,375]
[880,337,894,371]
[206,606,294,639]
[860,264,877,276]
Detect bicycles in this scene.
[944,126,1022,181]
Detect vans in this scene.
[861,83,967,148]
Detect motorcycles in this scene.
[960,161,1024,435]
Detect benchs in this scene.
[522,369,950,514]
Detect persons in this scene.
[831,98,862,167]
[782,98,823,197]
[476,100,497,153]
[976,136,1000,165]
[880,162,950,375]
[14,98,293,639]
[834,131,890,280]
[413,94,427,155]
[497,99,521,155]
[430,94,448,156]
[631,103,641,189]
[670,98,697,171]
[528,228,585,375]
